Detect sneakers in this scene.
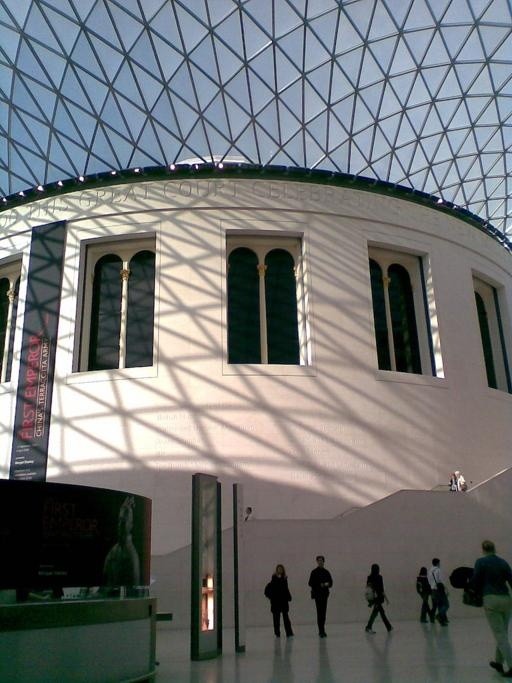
[502,668,512,678]
[489,660,503,672]
[274,631,328,639]
[365,626,377,634]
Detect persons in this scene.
[241,505,256,521]
[414,565,433,623]
[262,562,295,640]
[362,562,396,635]
[307,553,335,639]
[102,495,141,585]
[427,557,452,627]
[454,470,466,491]
[447,473,457,491]
[464,537,511,677]
